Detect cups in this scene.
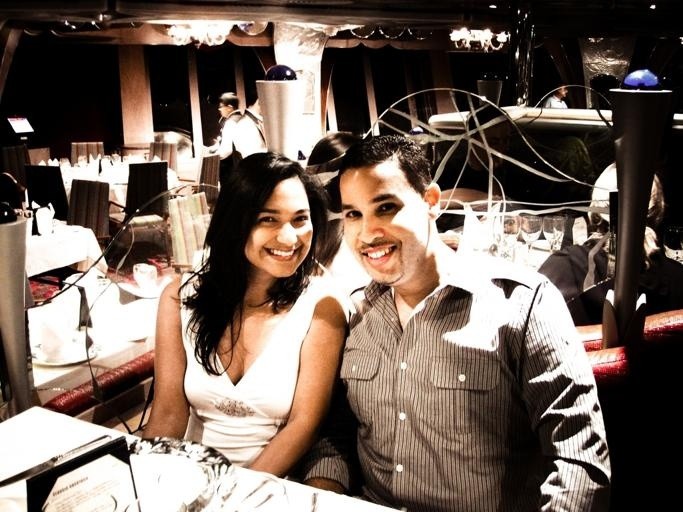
[58,153,149,174]
[131,263,158,290]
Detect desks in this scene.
[426,108,677,138]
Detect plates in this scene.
[32,349,97,368]
[126,450,211,512]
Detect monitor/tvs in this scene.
[7,116,35,134]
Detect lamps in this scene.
[264,60,316,94]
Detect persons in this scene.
[306,132,461,320]
[216,90,243,158]
[141,153,348,478]
[544,84,569,109]
[301,135,614,512]
[536,162,682,327]
[234,97,266,158]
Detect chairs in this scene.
[22,128,222,252]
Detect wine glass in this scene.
[542,215,565,256]
[491,215,521,260]
[519,216,543,255]
